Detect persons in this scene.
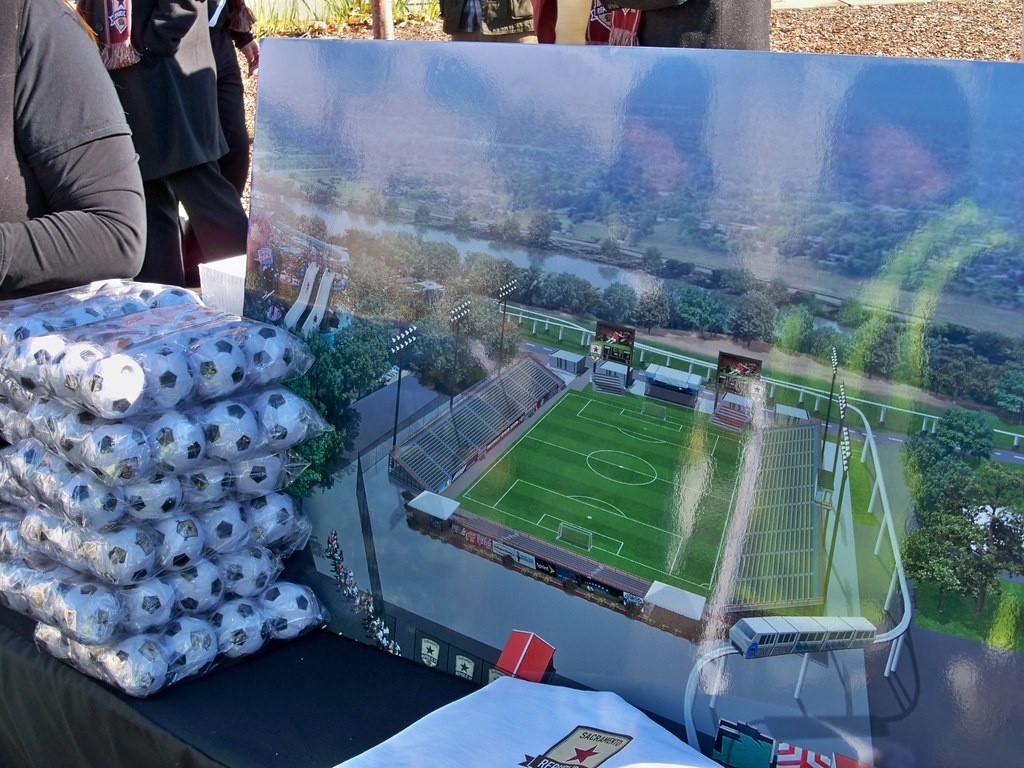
[769,65,1021,441]
[553,54,728,258]
[439,0,772,48]
[0,0,259,295]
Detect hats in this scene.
[842,67,972,181]
[623,56,713,161]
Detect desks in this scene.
[1,608,485,768]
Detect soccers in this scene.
[0,278,320,701]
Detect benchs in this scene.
[390,351,828,617]
